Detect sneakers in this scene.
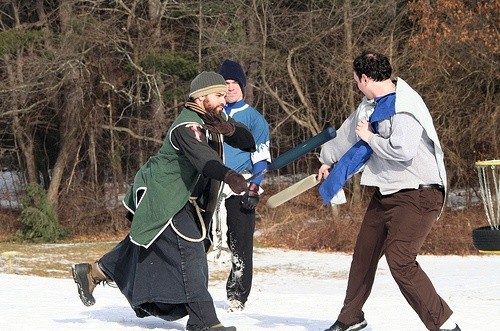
[324,318,368,331]
[72,262,106,306]
[435,323,461,331]
[186,321,236,331]
[227,301,244,312]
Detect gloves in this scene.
[240,182,259,209]
[202,109,235,139]
[224,170,247,194]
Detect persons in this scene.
[317,51,461,331]
[72,71,255,330]
[219,60,271,313]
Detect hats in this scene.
[219,59,246,87]
[189,70,228,100]
